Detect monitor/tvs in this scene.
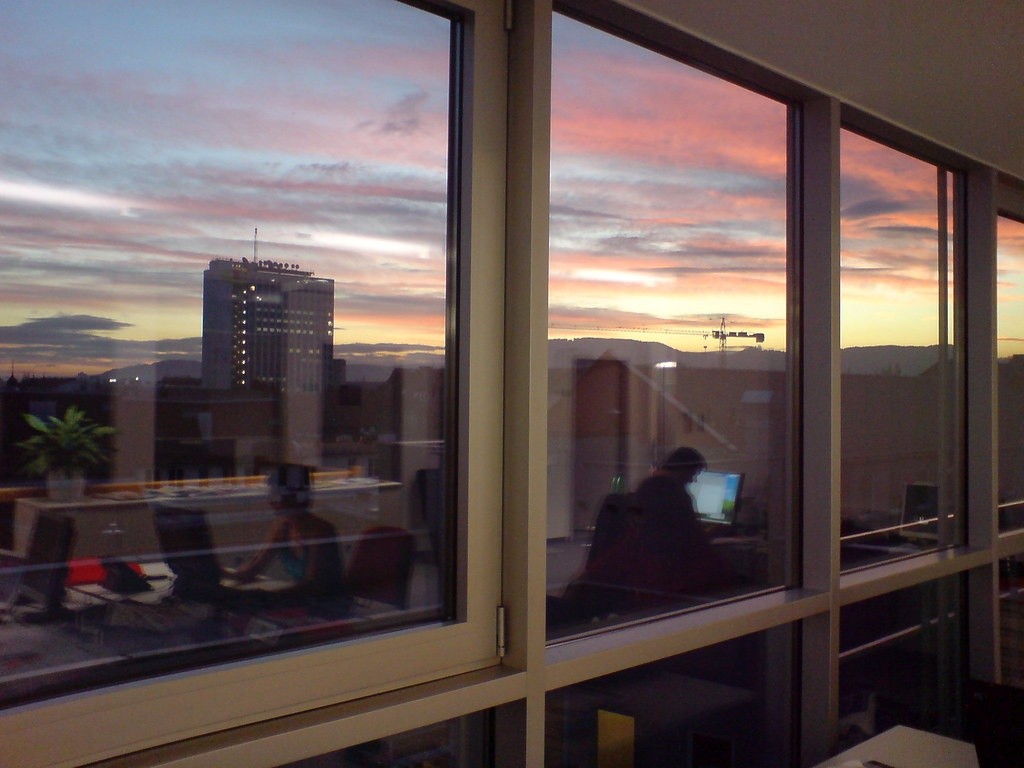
[17,509,77,608]
[688,470,744,527]
[899,481,940,545]
[148,501,222,586]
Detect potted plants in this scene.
[15,404,122,502]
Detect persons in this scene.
[225,465,343,601]
[638,449,725,564]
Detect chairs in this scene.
[588,492,685,594]
[340,527,419,610]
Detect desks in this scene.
[0,566,397,710]
[12,476,407,560]
